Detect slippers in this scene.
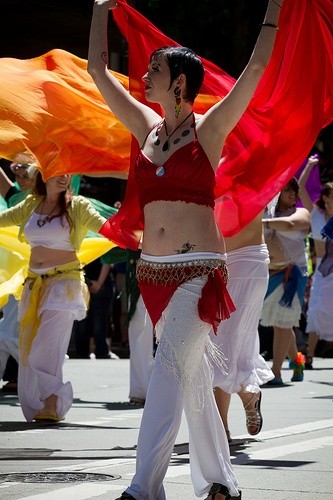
[32,410,65,422]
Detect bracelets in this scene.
[272,0,281,8]
[266,218,270,229]
[261,23,280,31]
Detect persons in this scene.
[0,154,333,444]
[86,0,283,500]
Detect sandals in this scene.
[244,391,263,436]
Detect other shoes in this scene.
[115,492,136,500]
[204,482,241,500]
[260,357,314,388]
[130,397,145,405]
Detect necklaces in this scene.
[154,110,193,153]
[37,198,58,228]
